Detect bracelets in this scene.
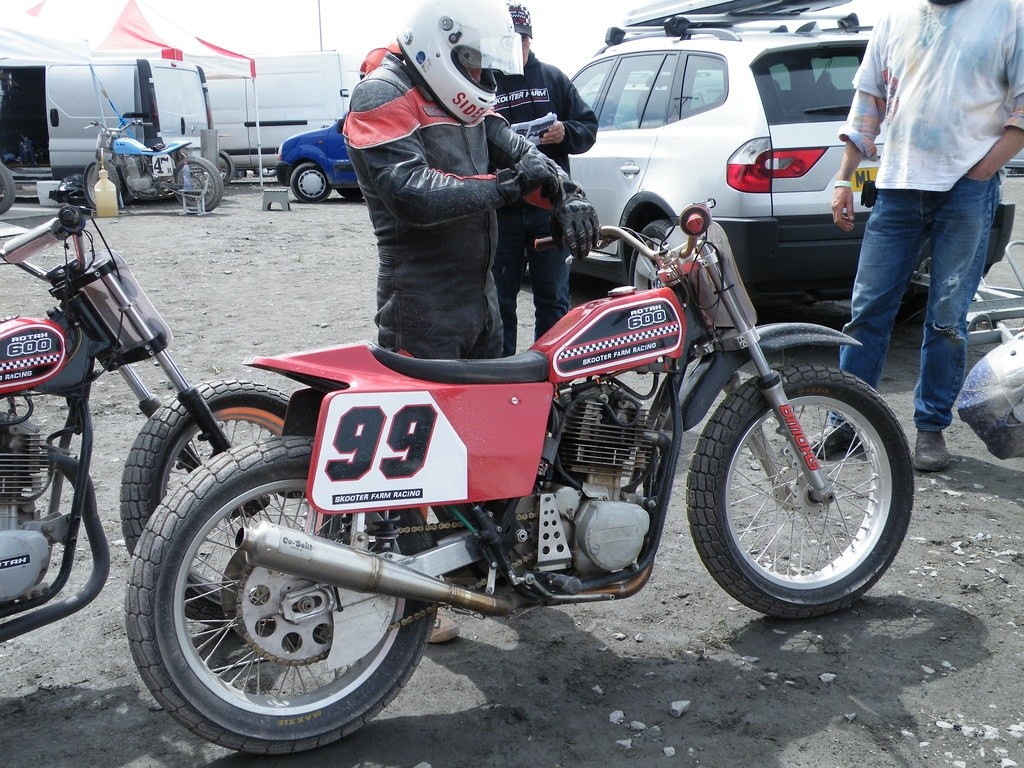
[833,181,853,189]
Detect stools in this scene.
[262,188,293,212]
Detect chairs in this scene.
[177,171,211,217]
[635,75,668,129]
[590,89,616,130]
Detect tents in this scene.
[0,0,267,185]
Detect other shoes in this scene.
[427,606,460,643]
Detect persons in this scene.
[346,0,602,641]
[812,0,1024,472]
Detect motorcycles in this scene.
[0,158,347,642]
[122,198,916,756]
[82,121,225,215]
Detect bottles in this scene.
[94,169,118,217]
[183,162,192,191]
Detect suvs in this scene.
[274,110,366,203]
[519,0,1015,320]
[576,67,724,126]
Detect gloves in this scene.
[491,148,559,204]
[549,191,600,259]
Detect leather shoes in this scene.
[806,423,864,458]
[914,428,949,470]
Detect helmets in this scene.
[396,0,514,125]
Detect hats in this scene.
[504,0,532,39]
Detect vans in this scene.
[0,57,216,201]
[193,49,364,185]
